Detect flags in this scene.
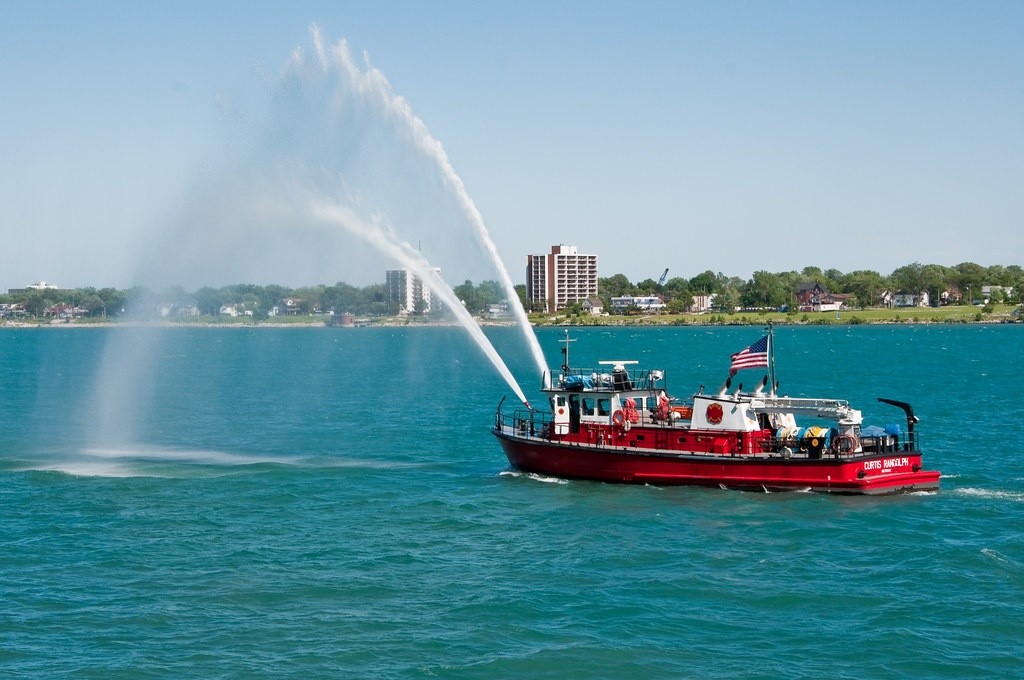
[729,334,769,377]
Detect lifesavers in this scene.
[613,410,626,427]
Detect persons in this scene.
[572,400,580,431]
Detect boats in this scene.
[491,321,940,494]
